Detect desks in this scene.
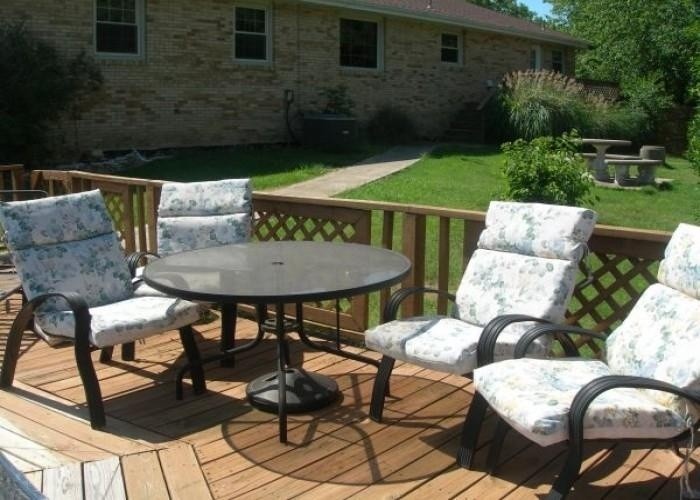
[570,134,631,184]
[143,238,416,414]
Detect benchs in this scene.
[581,149,659,182]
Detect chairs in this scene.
[100,178,256,367]
[366,204,594,472]
[472,223,700,500]
[1,190,201,432]
